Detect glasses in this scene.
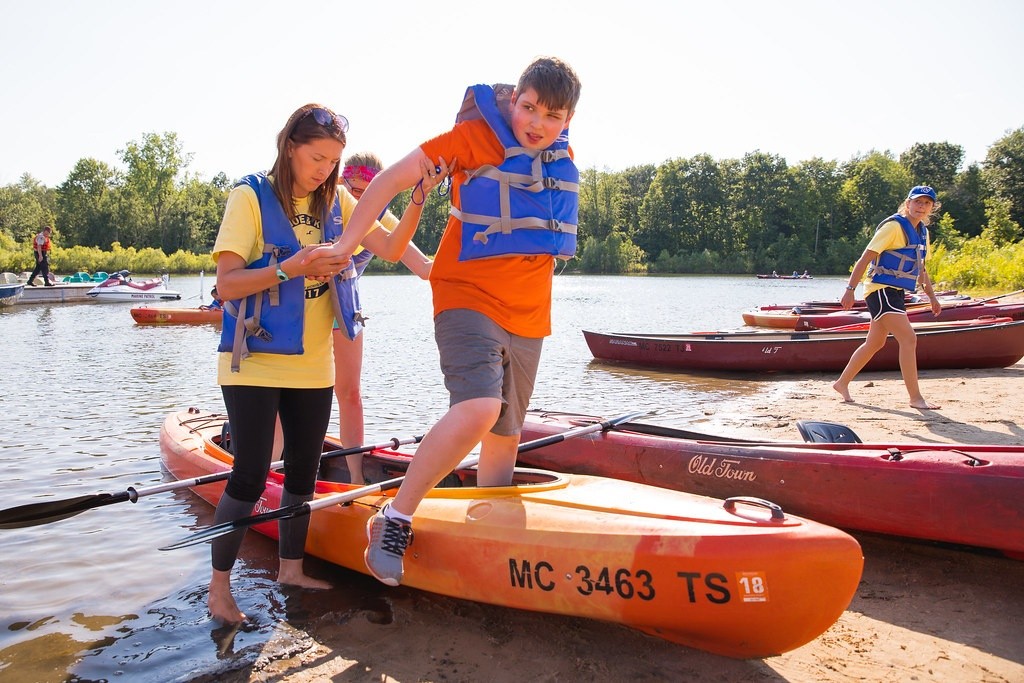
[344,178,365,197]
[294,108,349,133]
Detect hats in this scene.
[908,186,936,203]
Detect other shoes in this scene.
[44,283,56,286]
[26,281,37,287]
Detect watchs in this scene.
[845,285,856,290]
[276,262,289,282]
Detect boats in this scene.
[516,409,1024,560]
[742,290,1024,330]
[130,305,223,326]
[757,274,813,279]
[0,283,25,308]
[159,407,864,659]
[582,320,1024,373]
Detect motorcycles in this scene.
[86,270,182,302]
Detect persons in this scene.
[792,270,798,276]
[209,105,460,623]
[267,153,434,485]
[200,285,221,310]
[832,186,941,409]
[27,226,55,286]
[299,59,580,586]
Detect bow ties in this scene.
[363,504,414,587]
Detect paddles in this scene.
[159,405,658,553]
[979,290,1024,303]
[0,434,427,530]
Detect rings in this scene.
[330,270,333,276]
[431,174,437,179]
